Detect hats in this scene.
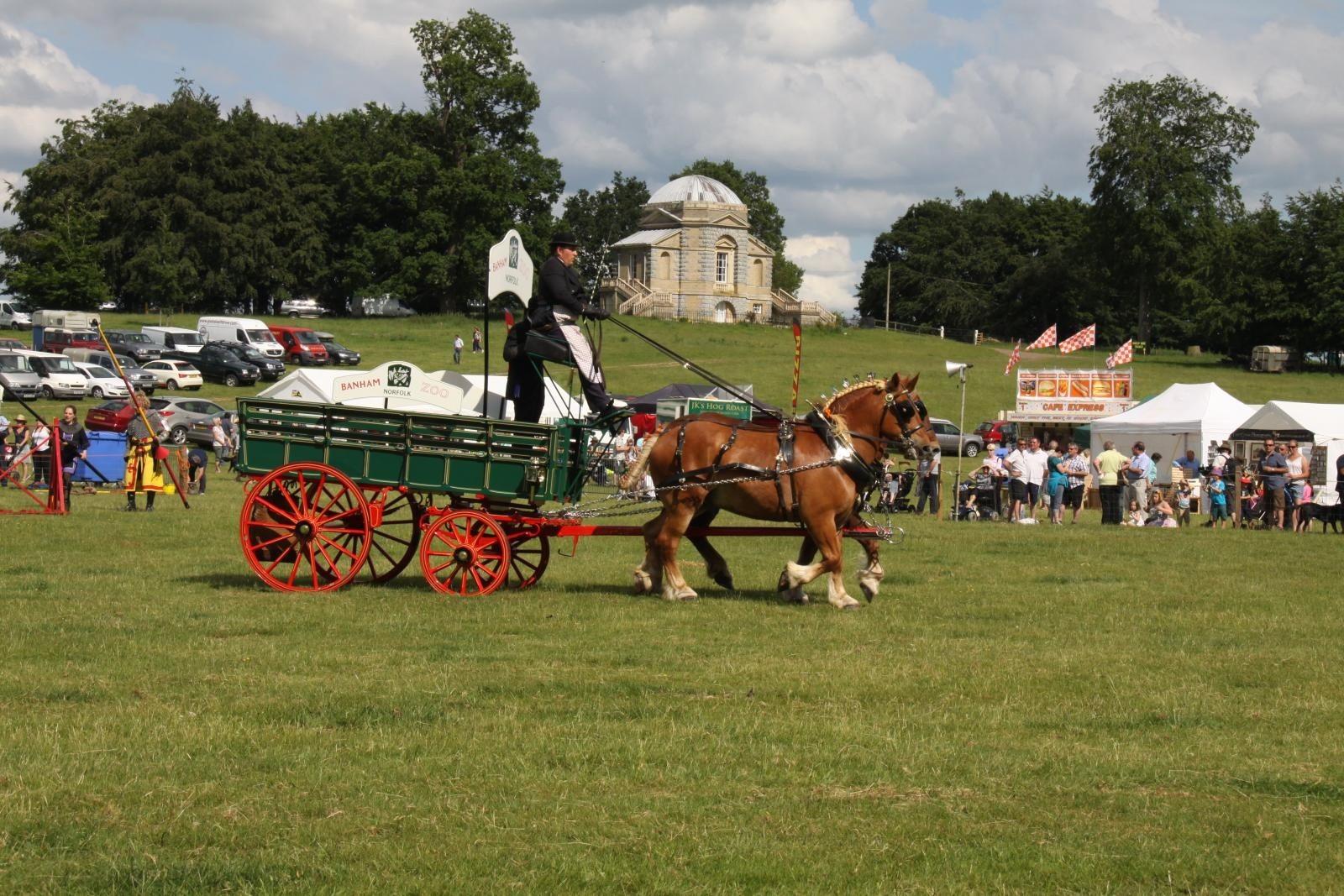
[13,414,27,424]
[550,233,580,246]
[1178,480,1188,485]
[1209,469,1223,477]
[190,454,201,465]
[1216,444,1232,455]
[0,416,10,431]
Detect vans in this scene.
[198,315,286,362]
[141,326,207,354]
[263,326,330,365]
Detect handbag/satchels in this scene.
[1119,460,1130,487]
[1146,517,1158,524]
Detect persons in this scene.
[587,428,656,502]
[188,450,208,496]
[503,298,545,459]
[0,406,91,511]
[453,334,464,364]
[124,390,170,511]
[879,436,1343,532]
[472,326,484,354]
[212,412,239,473]
[532,233,628,413]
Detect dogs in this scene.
[222,455,237,474]
[1291,502,1344,534]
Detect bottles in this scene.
[1116,381,1128,397]
[1241,517,1247,529]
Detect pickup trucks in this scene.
[161,344,265,387]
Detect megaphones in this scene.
[946,361,974,378]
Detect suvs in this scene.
[207,339,287,380]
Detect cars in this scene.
[975,419,1021,446]
[316,331,363,366]
[142,396,229,441]
[921,416,986,458]
[0,300,160,403]
[187,409,239,448]
[280,298,337,319]
[98,330,170,364]
[138,359,204,391]
[84,398,137,433]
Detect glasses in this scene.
[1018,443,1025,446]
[1067,448,1077,450]
[1277,448,1286,451]
[1264,444,1273,447]
[1288,446,1297,449]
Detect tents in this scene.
[256,361,589,424]
[1090,382,1256,514]
[1229,400,1344,506]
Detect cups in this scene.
[1254,519,1264,530]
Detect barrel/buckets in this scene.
[163,483,177,495]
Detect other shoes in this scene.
[123,504,135,511]
[198,491,205,495]
[146,505,154,511]
[601,406,629,417]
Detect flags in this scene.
[1106,338,1133,369]
[1058,324,1095,355]
[1004,339,1021,376]
[1026,324,1057,351]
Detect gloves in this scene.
[582,304,610,321]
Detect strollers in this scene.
[875,469,917,514]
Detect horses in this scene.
[634,371,940,609]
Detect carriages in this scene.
[229,370,945,613]
